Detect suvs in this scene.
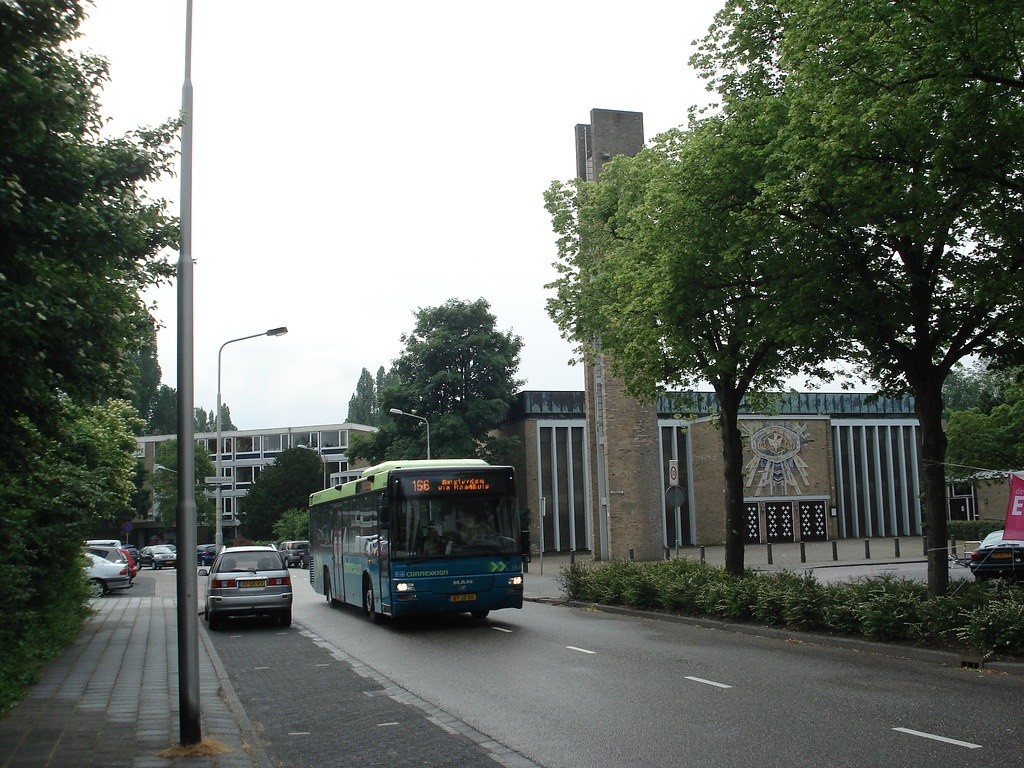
[198,544,293,631]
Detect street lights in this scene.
[297,444,326,490]
[390,409,430,459]
[215,326,288,557]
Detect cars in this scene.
[139,546,176,570]
[84,540,140,578]
[298,550,309,569]
[82,553,130,598]
[164,545,177,554]
[82,547,133,594]
[278,540,309,568]
[365,538,388,556]
[197,544,216,567]
[970,530,1024,581]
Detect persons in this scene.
[423,529,448,555]
[462,509,495,544]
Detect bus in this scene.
[308,459,531,624]
